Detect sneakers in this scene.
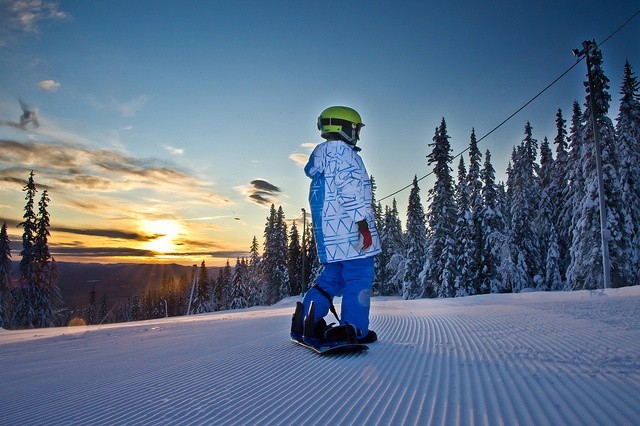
[356,328,378,343]
[292,318,332,340]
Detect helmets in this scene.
[317,107,366,147]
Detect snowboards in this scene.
[291,332,369,354]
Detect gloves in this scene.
[355,219,371,250]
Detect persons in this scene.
[288,103,383,346]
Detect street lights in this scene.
[302,209,305,296]
[193,265,198,296]
